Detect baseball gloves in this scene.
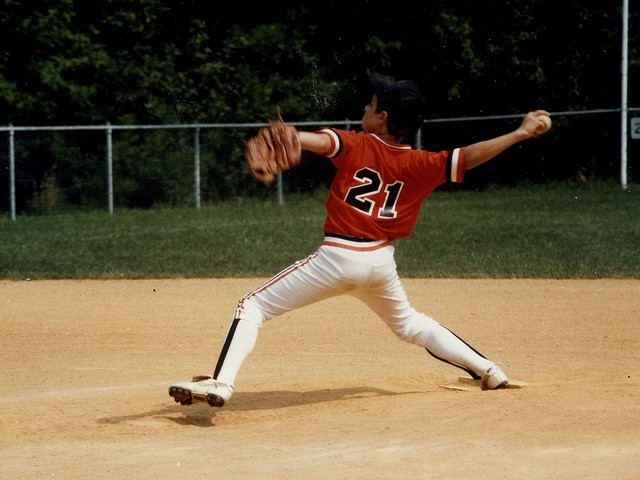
[246,122,301,184]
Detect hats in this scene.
[370,73,426,132]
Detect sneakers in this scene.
[483,367,506,387]
[169,379,234,406]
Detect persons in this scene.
[168,81,551,408]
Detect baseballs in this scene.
[535,116,552,135]
